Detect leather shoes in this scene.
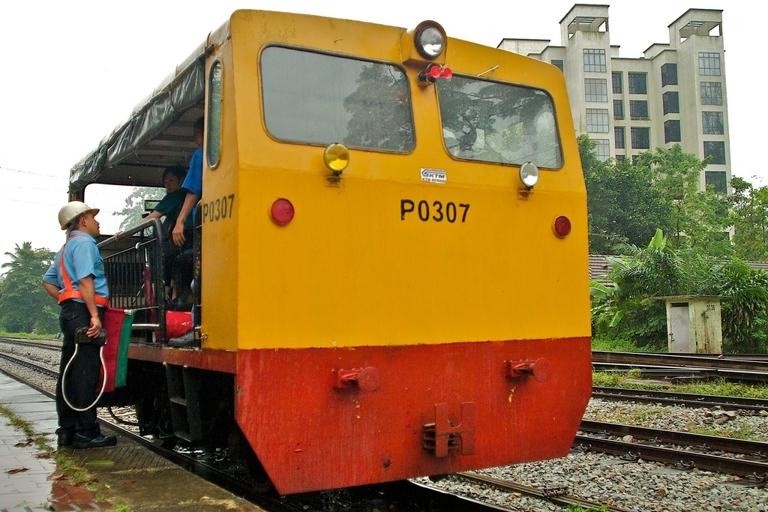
[57,426,117,449]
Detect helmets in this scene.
[59,201,99,230]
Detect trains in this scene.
[68,9,592,496]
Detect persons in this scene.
[114,166,188,304]
[168,116,204,348]
[41,201,118,448]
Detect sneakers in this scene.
[168,330,199,347]
